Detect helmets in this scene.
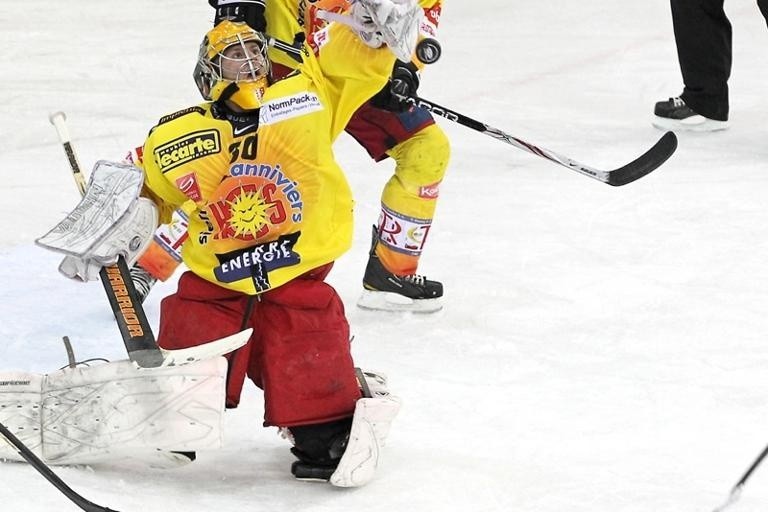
[192,19,272,111]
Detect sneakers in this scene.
[361,224,444,300]
[654,97,699,120]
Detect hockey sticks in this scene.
[0,424,120,512]
[710,449,766,512]
[263,33,677,187]
[48,110,253,371]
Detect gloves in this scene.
[387,58,419,110]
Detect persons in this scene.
[32,1,438,491]
[638,0,768,138]
[209,0,446,303]
